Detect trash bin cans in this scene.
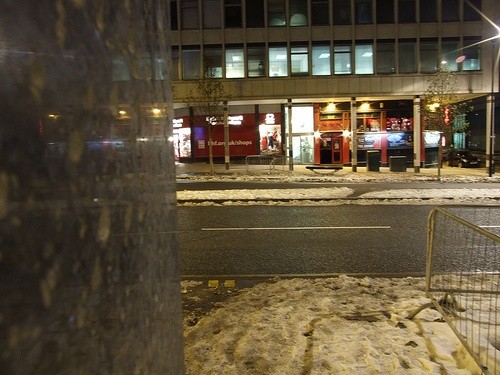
[367,150,379,171]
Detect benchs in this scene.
[306,164,343,174]
